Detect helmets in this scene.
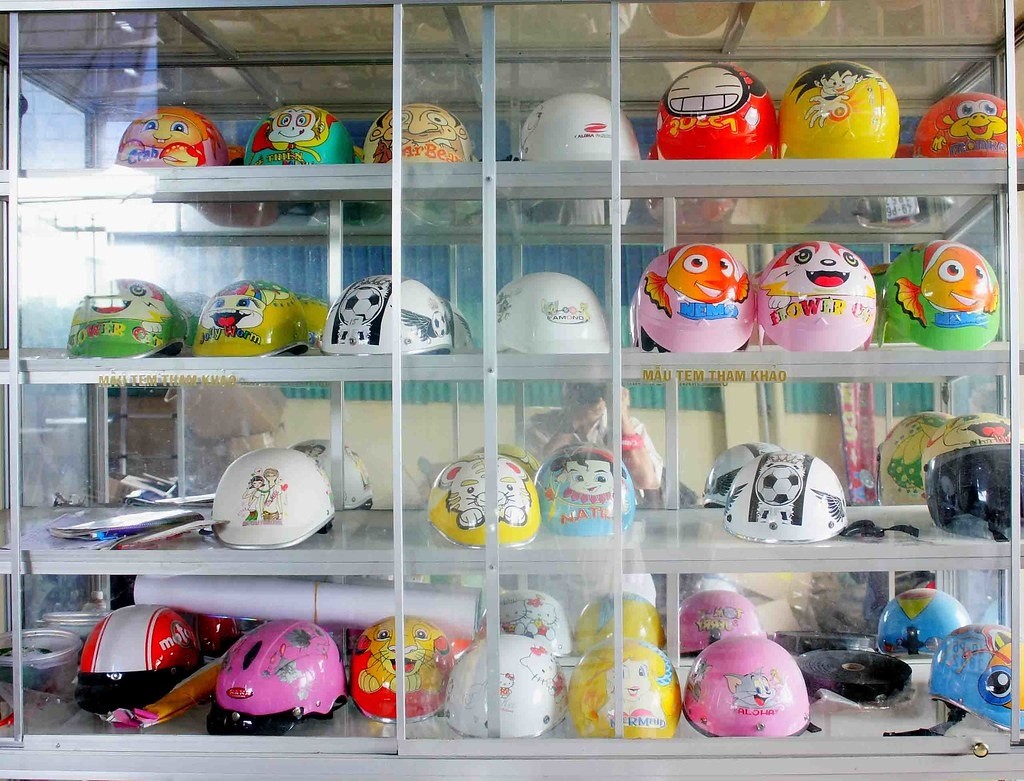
[758,240,877,352]
[911,93,1024,159]
[195,279,310,359]
[316,273,455,355]
[780,59,904,159]
[111,106,229,169]
[363,104,478,164]
[635,241,759,350]
[72,409,1017,743]
[62,278,187,361]
[871,236,1004,349]
[517,92,642,162]
[493,271,622,351]
[654,64,782,158]
[242,105,355,166]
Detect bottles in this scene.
[83,589,106,612]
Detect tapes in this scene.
[773,630,876,655]
[796,648,913,704]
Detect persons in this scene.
[518,360,665,621]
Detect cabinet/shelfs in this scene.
[0,12,1023,758]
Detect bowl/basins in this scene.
[0,631,81,692]
[38,611,110,664]
